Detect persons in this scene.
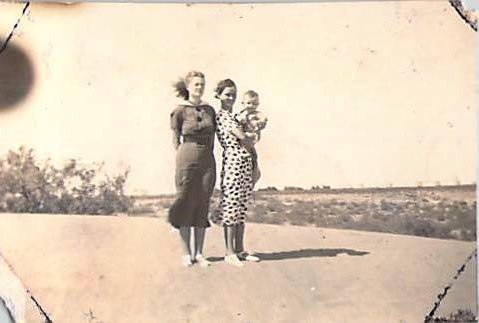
[233,90,268,187]
[211,78,261,265]
[167,72,217,268]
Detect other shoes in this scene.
[195,255,212,267]
[225,254,245,267]
[183,253,193,268]
[237,251,260,262]
